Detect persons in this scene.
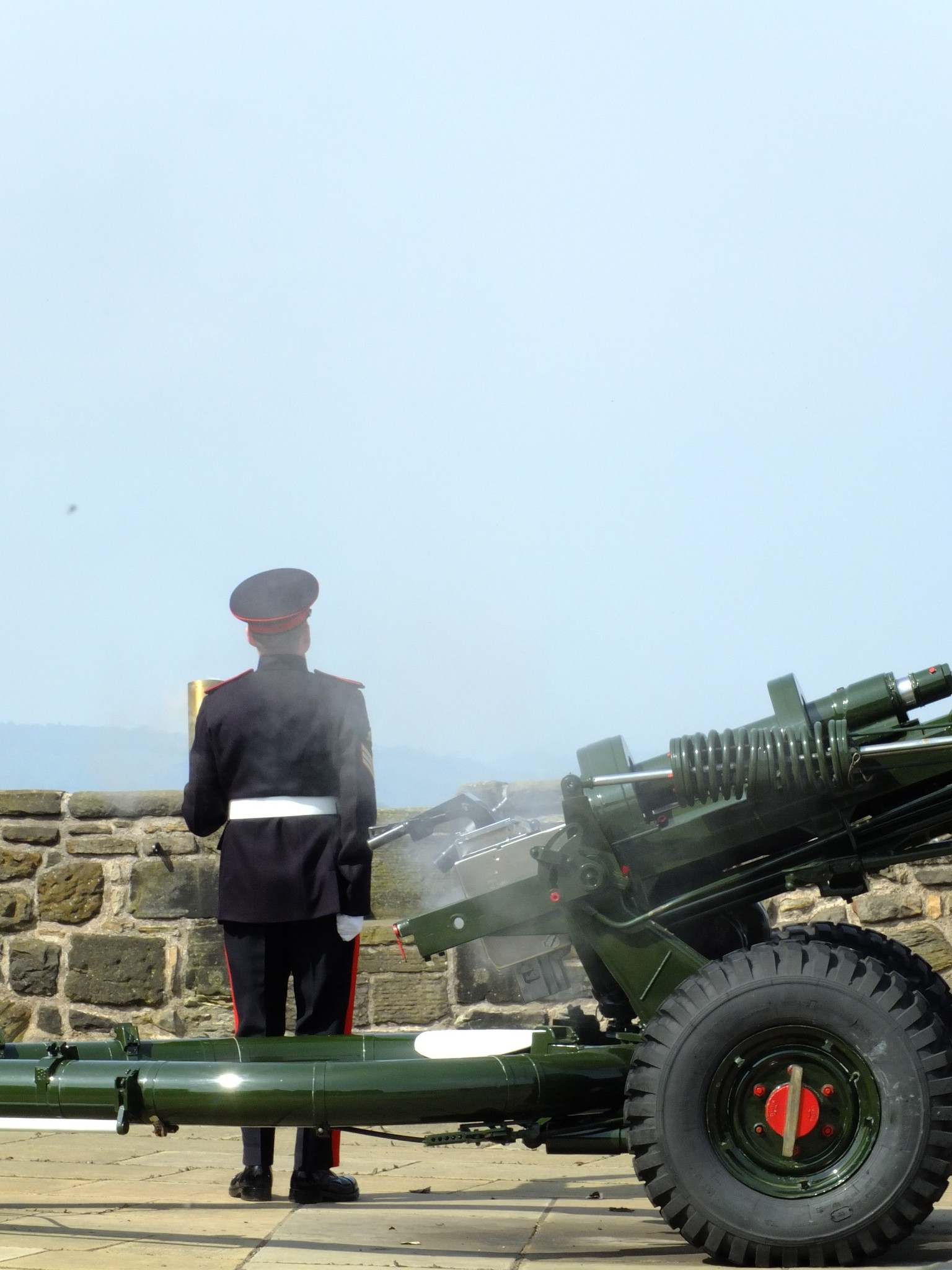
[183,569,377,1204]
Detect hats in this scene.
[229,568,320,634]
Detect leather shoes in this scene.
[289,1169,360,1205]
[228,1165,273,1202]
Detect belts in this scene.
[228,796,339,820]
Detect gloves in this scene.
[336,914,364,942]
